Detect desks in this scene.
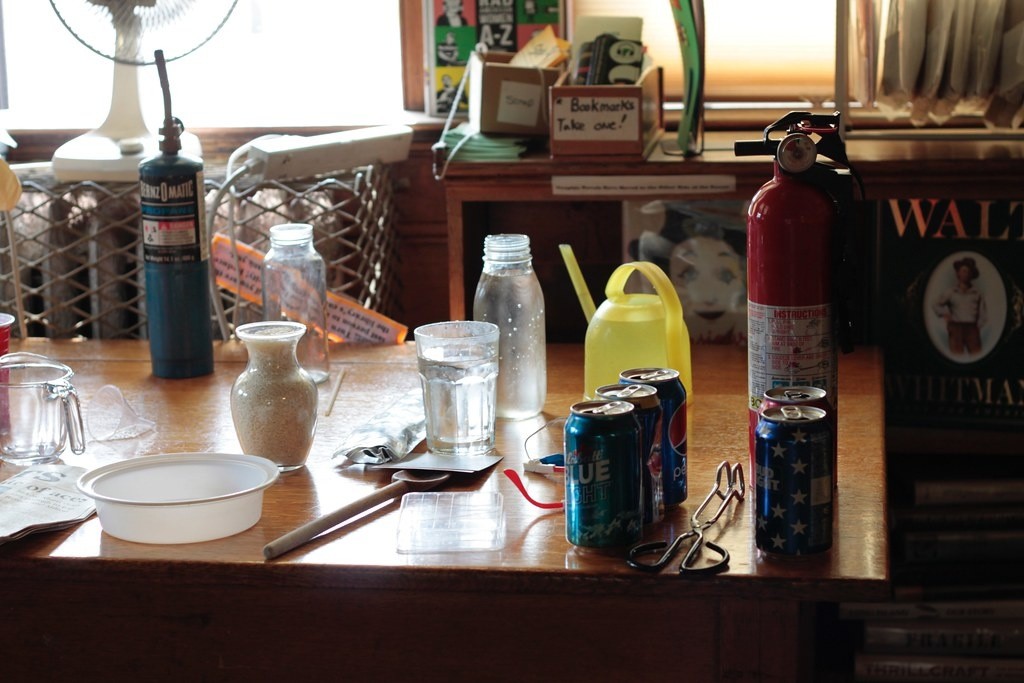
[0,337,886,683]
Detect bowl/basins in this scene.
[73,452,280,545]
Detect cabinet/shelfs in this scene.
[431,130,1024,683]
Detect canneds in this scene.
[562,367,687,553]
[752,386,835,560]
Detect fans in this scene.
[48,0,238,182]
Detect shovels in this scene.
[260,471,453,560]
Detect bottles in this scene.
[472,233,548,420]
[228,320,320,473]
[260,222,330,386]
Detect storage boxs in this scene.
[468,50,560,134]
[546,63,666,163]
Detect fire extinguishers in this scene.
[733,112,868,495]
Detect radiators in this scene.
[0,189,347,337]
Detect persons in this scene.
[935,258,988,355]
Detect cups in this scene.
[0,311,16,434]
[413,321,501,458]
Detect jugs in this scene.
[0,351,84,465]
[558,242,693,401]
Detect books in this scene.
[855,200,1024,683]
